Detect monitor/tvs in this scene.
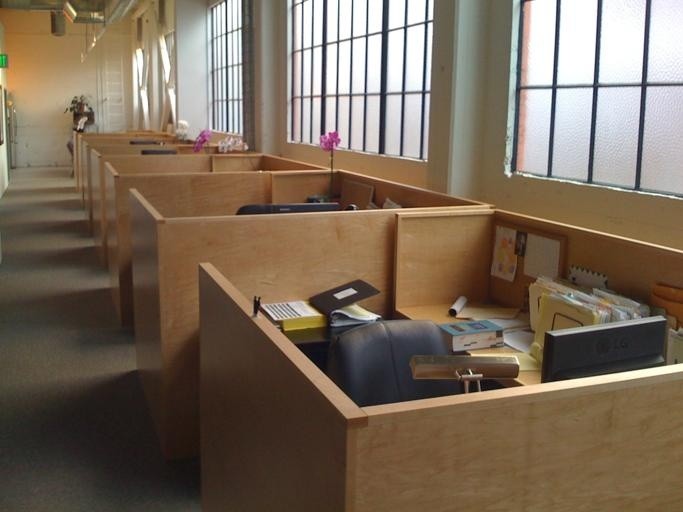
[541,315,668,384]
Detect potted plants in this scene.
[63,95,95,126]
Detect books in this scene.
[437,319,504,353]
[260,279,381,331]
[529,276,683,365]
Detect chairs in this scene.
[328,317,465,404]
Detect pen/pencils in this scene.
[504,324,529,333]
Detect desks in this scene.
[395,300,544,388]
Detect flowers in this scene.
[192,130,248,155]
[318,131,343,203]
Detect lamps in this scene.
[407,352,521,393]
